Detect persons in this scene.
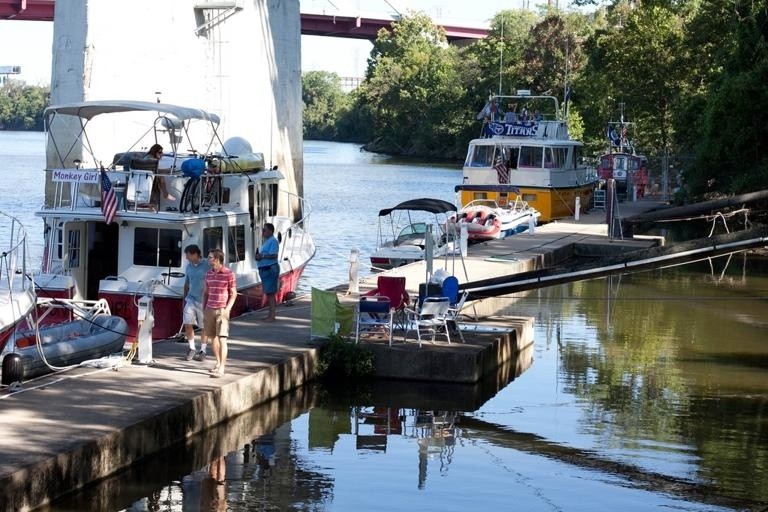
[201,249,238,377]
[181,245,211,361]
[144,144,176,203]
[484,116,491,122]
[505,107,516,122]
[254,222,280,322]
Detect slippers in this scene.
[209,372,224,378]
[265,315,275,321]
[209,367,219,372]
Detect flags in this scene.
[100,165,119,225]
[621,124,628,140]
[565,84,572,115]
[608,125,621,146]
[496,148,508,184]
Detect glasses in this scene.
[208,255,216,259]
[262,228,269,232]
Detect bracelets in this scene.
[262,254,264,259]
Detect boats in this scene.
[0,314,130,384]
[0,211,40,354]
[35,99,318,352]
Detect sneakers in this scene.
[197,351,207,361]
[185,349,196,361]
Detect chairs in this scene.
[126,157,160,214]
[310,277,470,349]
